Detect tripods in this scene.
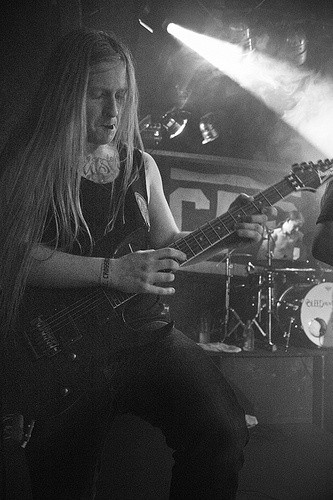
[216,228,303,352]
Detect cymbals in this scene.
[208,253,253,264]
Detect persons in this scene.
[267,180,333,269]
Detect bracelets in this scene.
[100,258,111,289]
[0,28,278,500]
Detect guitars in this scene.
[0,159,333,420]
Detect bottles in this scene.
[241,319,254,352]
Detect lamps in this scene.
[227,23,256,57]
[161,108,193,141]
[199,112,221,145]
[283,29,308,67]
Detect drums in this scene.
[276,281,332,349]
[254,226,309,269]
